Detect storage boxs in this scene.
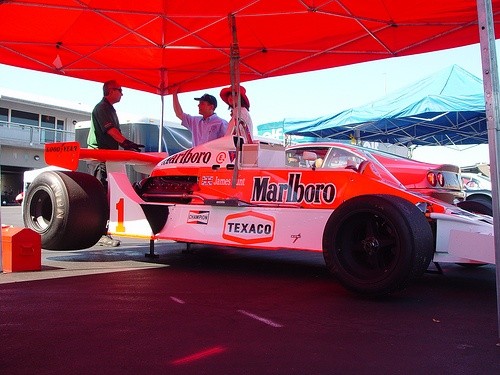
[1,225,42,273]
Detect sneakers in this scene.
[96,235,120,247]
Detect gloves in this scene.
[119,139,145,152]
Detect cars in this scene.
[283,142,465,206]
[458,172,493,218]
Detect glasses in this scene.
[112,88,122,92]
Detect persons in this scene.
[86,80,146,246]
[219,84,254,140]
[173,88,228,147]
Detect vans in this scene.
[74,119,194,160]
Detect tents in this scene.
[282,61,488,148]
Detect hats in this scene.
[220,86,249,108]
[194,94,217,106]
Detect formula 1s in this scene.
[21,117,496,298]
